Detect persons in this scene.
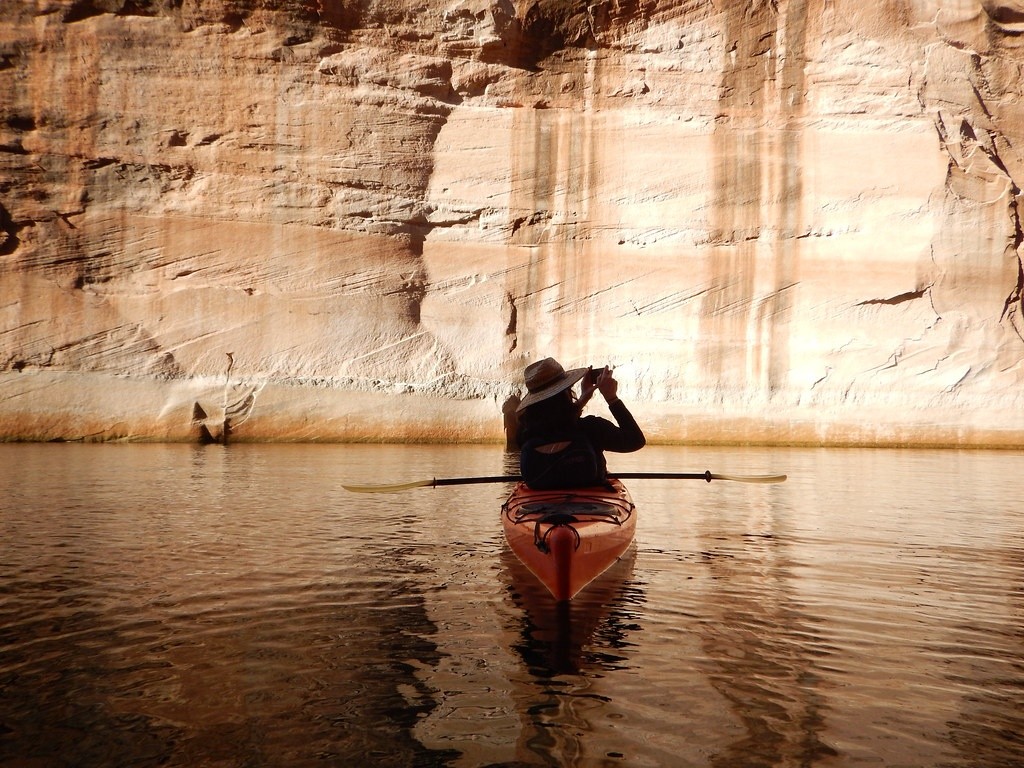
[515,357,646,492]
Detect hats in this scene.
[516,360,588,413]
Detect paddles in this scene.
[340,472,788,495]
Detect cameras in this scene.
[590,368,605,384]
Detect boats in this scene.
[498,472,638,602]
